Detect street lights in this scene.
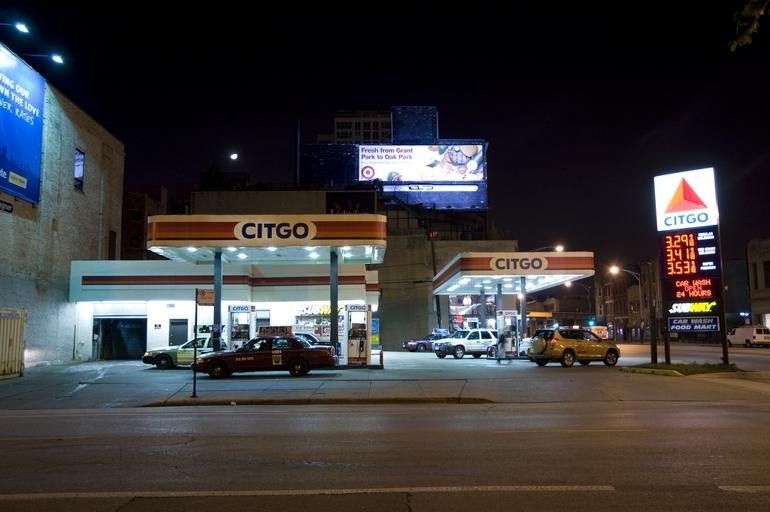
[567,281,592,313]
[533,246,562,251]
[613,268,643,318]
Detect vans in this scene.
[727,326,770,348]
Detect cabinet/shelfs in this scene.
[295,315,344,365]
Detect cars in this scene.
[519,327,620,367]
[143,332,341,378]
[403,329,498,359]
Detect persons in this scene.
[497,333,512,364]
